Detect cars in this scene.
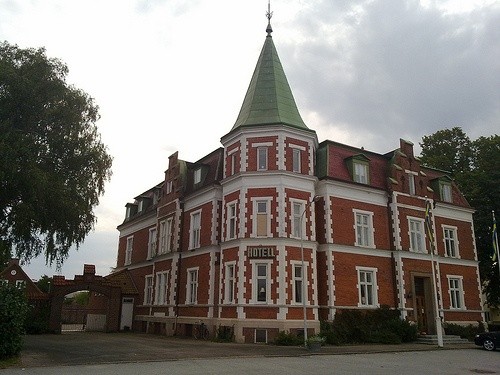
[475,331,500,352]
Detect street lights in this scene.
[301,194,321,352]
